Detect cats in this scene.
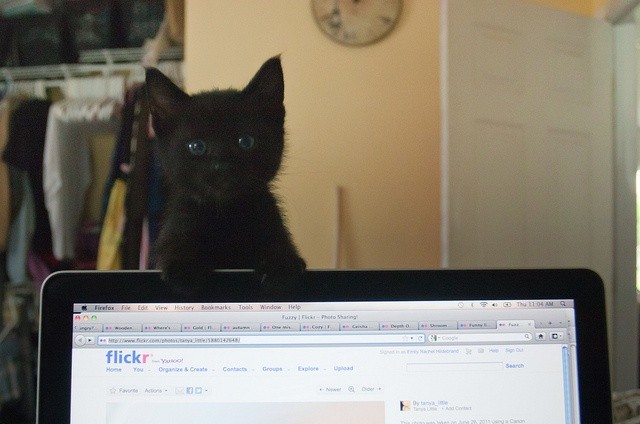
[145,53,307,297]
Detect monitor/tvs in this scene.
[37,270,614,424]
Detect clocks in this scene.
[310,0,403,48]
